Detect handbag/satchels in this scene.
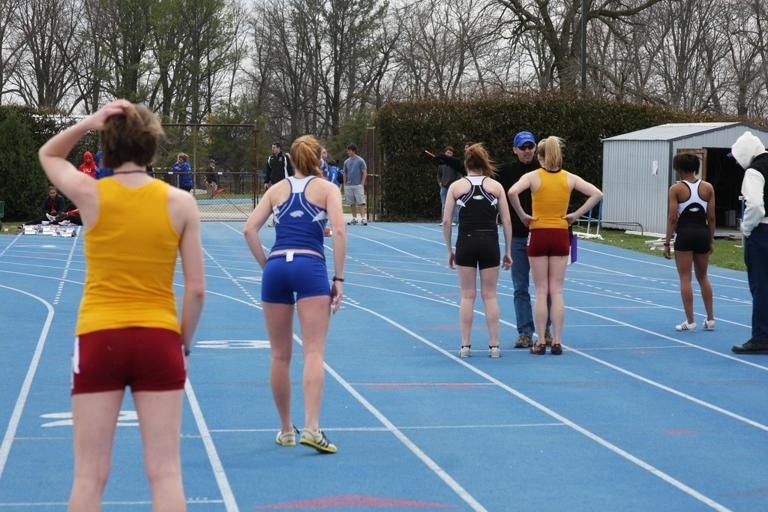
[337,168,345,183]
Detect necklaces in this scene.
[110,170,148,175]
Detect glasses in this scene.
[518,144,534,150]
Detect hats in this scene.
[514,131,535,147]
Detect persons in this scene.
[44,186,83,226]
[507,135,605,355]
[36,98,206,512]
[264,143,293,191]
[80,151,109,180]
[436,145,462,227]
[727,131,768,354]
[319,143,366,226]
[662,152,716,331]
[172,153,192,192]
[430,141,474,178]
[491,131,574,348]
[442,139,513,360]
[240,134,345,454]
[206,159,218,198]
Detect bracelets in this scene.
[664,243,670,247]
[184,349,189,356]
[332,276,344,282]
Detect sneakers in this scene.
[440,222,457,226]
[488,345,502,358]
[703,318,716,330]
[276,431,297,447]
[41,213,56,226]
[268,221,276,226]
[517,338,562,354]
[348,218,368,226]
[675,320,697,331]
[299,428,337,453]
[732,340,768,354]
[459,347,472,358]
